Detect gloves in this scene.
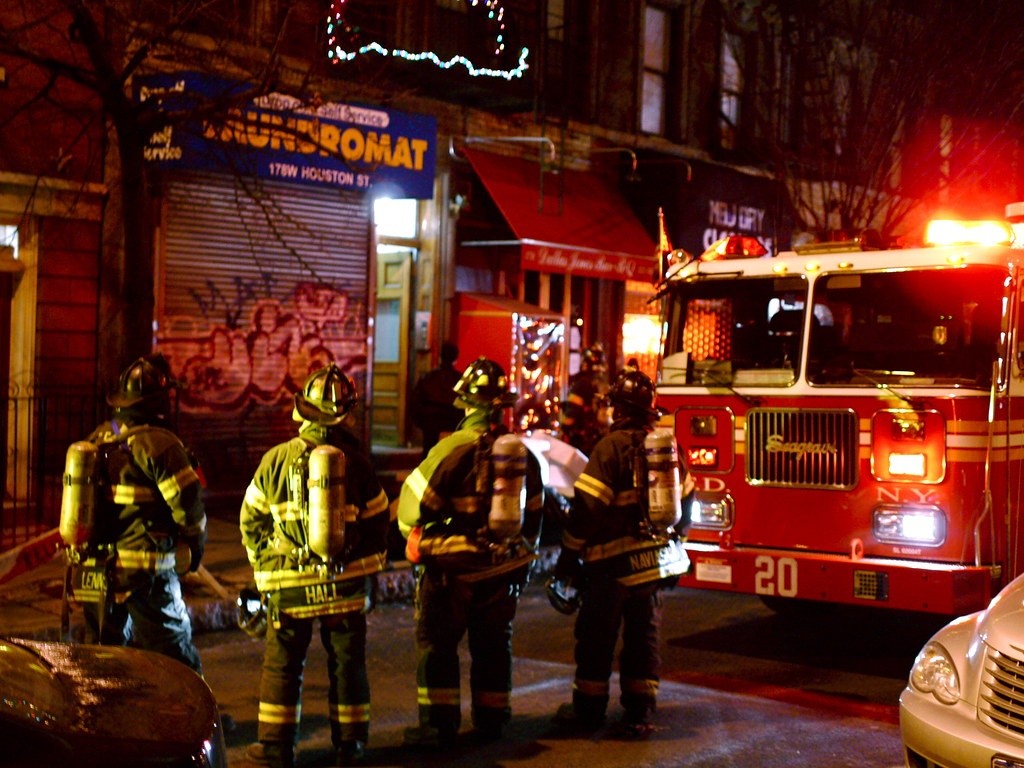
[662,575,679,591]
[184,532,207,572]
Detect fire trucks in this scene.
[647,219,1024,615]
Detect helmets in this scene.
[106,352,171,407]
[605,371,663,418]
[453,357,520,403]
[294,362,359,424]
[582,343,606,365]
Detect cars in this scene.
[0,635,229,768]
[896,572,1024,768]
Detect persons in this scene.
[59,354,235,734]
[397,360,545,747]
[239,367,392,765]
[415,341,464,458]
[553,342,615,455]
[553,371,696,732]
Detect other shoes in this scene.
[479,725,505,739]
[624,708,652,724]
[558,703,608,725]
[403,726,460,747]
[246,742,296,766]
[336,740,364,768]
